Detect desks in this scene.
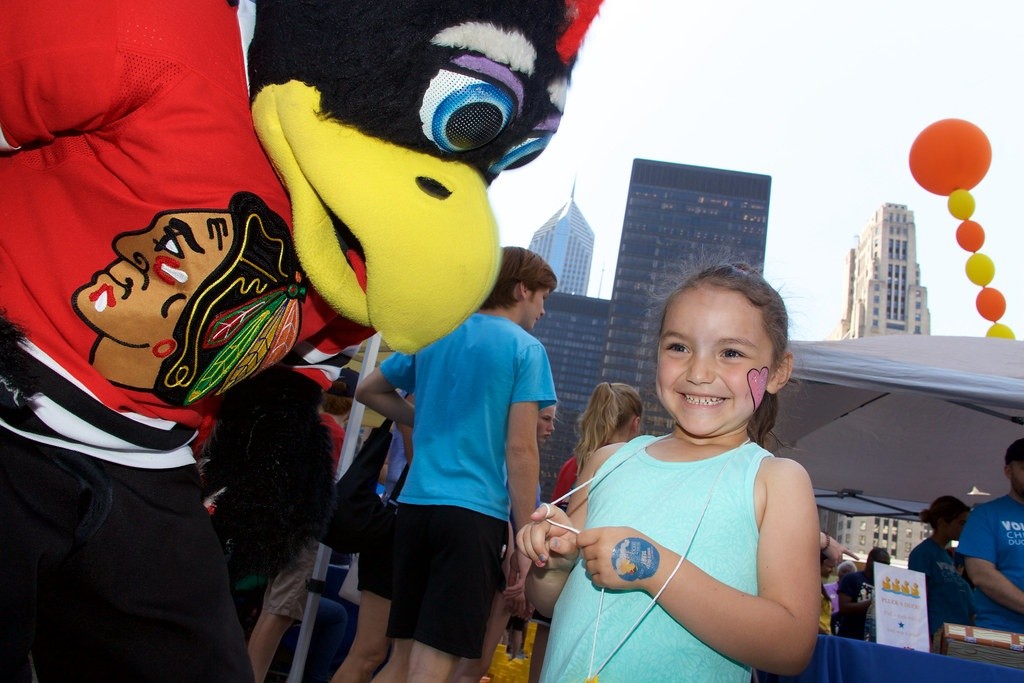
[745,635,1023,683]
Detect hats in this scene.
[325,366,360,396]
[1004,436,1024,463]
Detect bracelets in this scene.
[821,533,830,551]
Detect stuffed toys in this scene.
[0,0,602,683]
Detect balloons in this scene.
[966,253,995,287]
[948,189,975,220]
[909,118,992,195]
[976,288,1006,323]
[956,220,985,253]
[986,324,1016,340]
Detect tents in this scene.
[768,333,1024,522]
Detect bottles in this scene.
[864,598,875,643]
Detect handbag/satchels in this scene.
[309,426,397,553]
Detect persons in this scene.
[247,367,414,683]
[819,495,977,643]
[516,265,820,683]
[355,247,557,683]
[954,437,1024,634]
[451,382,643,683]
